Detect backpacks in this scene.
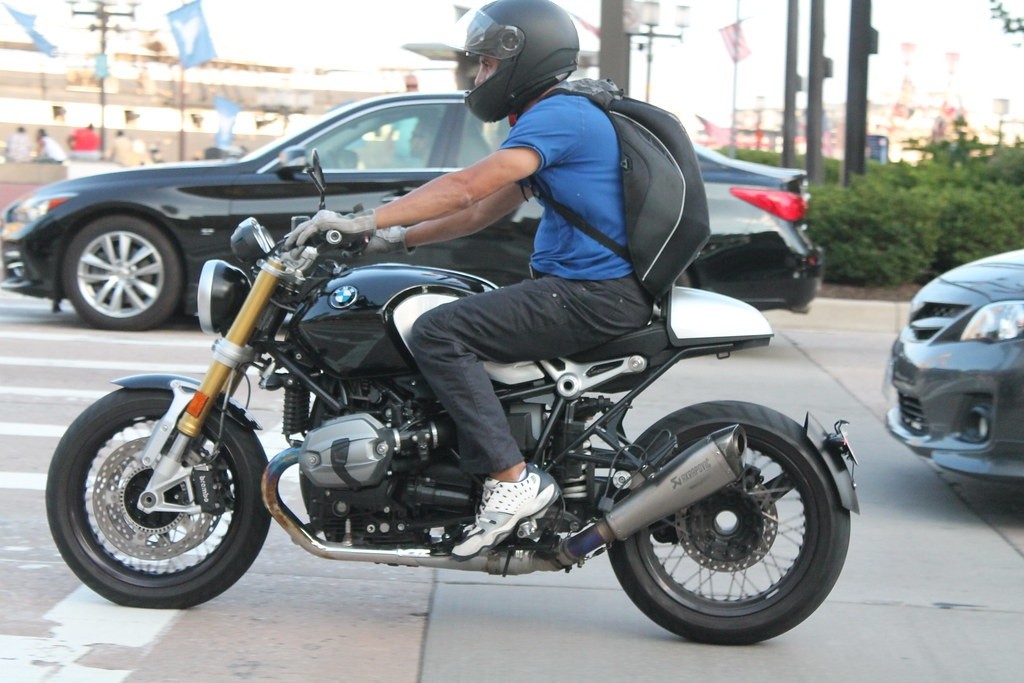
[528,74,711,301]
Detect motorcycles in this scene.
[44,143,862,646]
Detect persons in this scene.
[32,128,66,162]
[285,1,652,561]
[111,131,136,167]
[5,127,31,161]
[325,114,438,169]
[68,125,100,161]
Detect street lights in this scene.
[625,1,692,104]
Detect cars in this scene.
[1,88,827,331]
[878,248,1023,484]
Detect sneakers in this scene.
[451,462,562,561]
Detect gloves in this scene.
[285,210,376,246]
[366,225,414,254]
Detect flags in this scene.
[167,0,217,69]
[7,6,56,57]
[215,98,239,148]
[721,24,751,63]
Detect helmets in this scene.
[459,0,581,123]
